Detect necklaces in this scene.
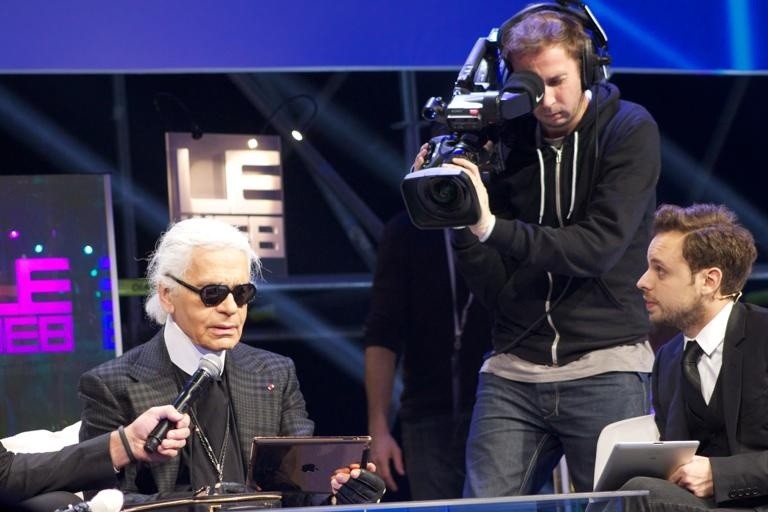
[190,411,230,483]
[187,402,230,470]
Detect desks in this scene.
[264,489,650,512]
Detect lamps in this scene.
[255,95,318,142]
[147,87,202,140]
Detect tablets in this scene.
[586,439,700,512]
[246,435,372,493]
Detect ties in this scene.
[680,340,716,457]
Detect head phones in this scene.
[498,1,610,91]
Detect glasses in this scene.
[167,272,255,307]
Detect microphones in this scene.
[144,353,221,454]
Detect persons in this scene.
[361,204,496,502]
[0,403,194,512]
[77,214,391,508]
[410,0,664,502]
[599,201,767,512]
